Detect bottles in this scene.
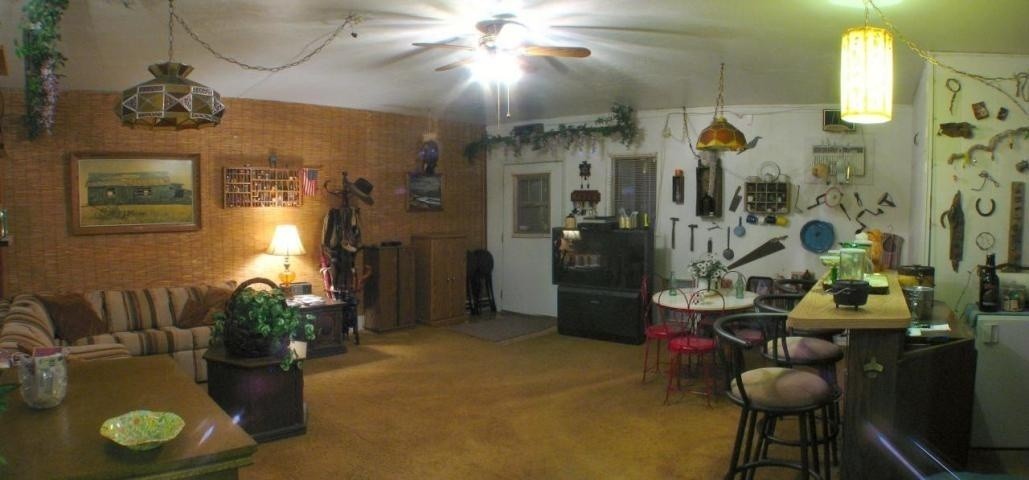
[617,207,651,230]
[980,253,1000,312]
[735,273,745,298]
[830,263,837,285]
[669,269,678,297]
[845,162,855,185]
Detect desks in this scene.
[787,262,910,479]
[202,344,308,444]
[0,354,258,480]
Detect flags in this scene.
[300,167,320,197]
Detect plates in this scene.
[99,409,187,453]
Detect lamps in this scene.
[695,60,747,152]
[112,1,223,132]
[839,0,894,126]
[265,225,306,300]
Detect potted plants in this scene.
[209,286,316,370]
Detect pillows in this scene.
[178,298,210,329]
[202,284,232,324]
[40,294,105,343]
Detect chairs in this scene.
[714,312,838,479]
[466,249,496,316]
[639,273,773,409]
[753,278,843,464]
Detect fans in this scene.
[411,21,591,73]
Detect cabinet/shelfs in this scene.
[558,285,651,345]
[894,300,975,476]
[362,243,417,333]
[221,167,304,208]
[412,233,467,326]
[744,182,789,214]
[696,167,722,216]
[284,294,346,358]
[279,282,311,294]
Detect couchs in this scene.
[0,281,231,385]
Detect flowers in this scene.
[687,255,726,281]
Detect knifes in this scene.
[729,185,743,213]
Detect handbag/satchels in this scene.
[341,211,362,253]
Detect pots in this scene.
[799,220,835,255]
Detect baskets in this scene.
[223,278,290,357]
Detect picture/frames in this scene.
[404,172,449,213]
[69,150,201,236]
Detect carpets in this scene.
[447,316,557,344]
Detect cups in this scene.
[839,247,867,282]
[746,194,790,226]
[11,350,70,411]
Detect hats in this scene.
[350,178,373,200]
